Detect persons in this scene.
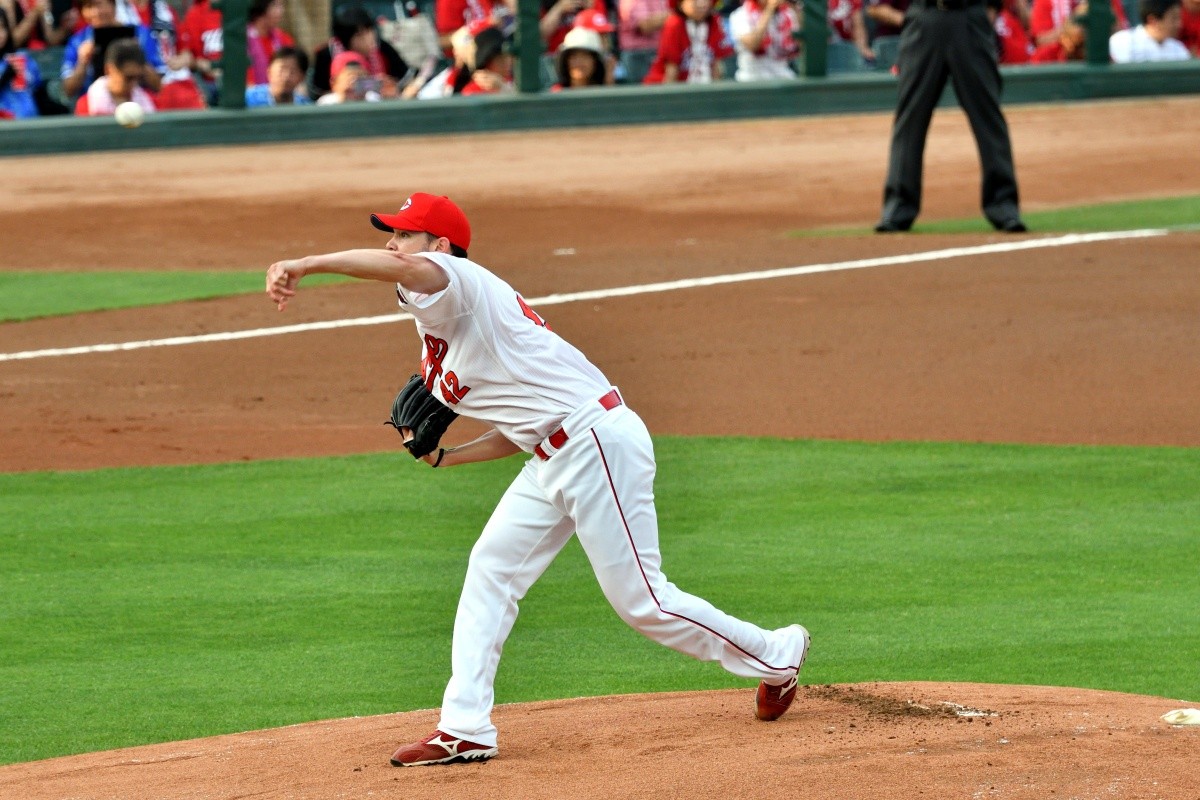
[0,1,1200,120]
[265,192,810,766]
[863,1,1030,234]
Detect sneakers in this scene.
[753,625,812,721]
[389,728,499,765]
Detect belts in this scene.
[532,390,621,461]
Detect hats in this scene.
[574,9,615,33]
[370,192,471,251]
[329,50,366,78]
[558,28,601,55]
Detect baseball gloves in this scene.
[391,374,461,461]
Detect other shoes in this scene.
[873,220,903,232]
[993,217,1027,233]
